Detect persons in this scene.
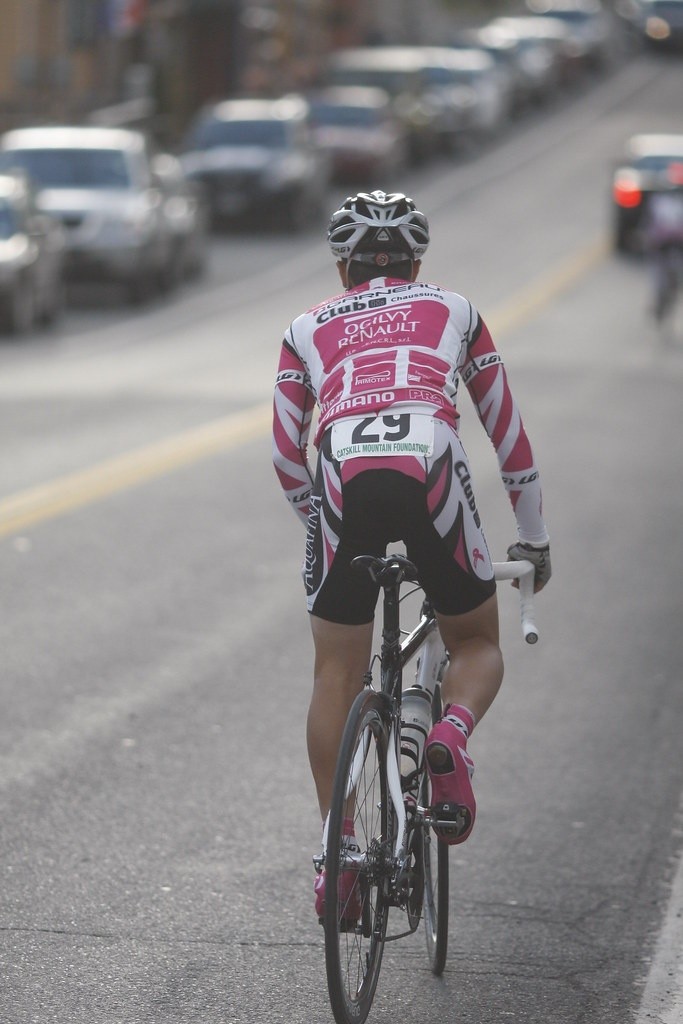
[269,191,550,923]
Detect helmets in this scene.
[326,190,430,266]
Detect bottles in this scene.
[399,684,433,776]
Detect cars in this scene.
[1,0,682,342]
[612,135,683,256]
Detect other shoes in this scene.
[312,864,361,919]
[424,718,476,845]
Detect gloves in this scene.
[507,539,552,594]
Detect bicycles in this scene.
[312,551,544,1024]
